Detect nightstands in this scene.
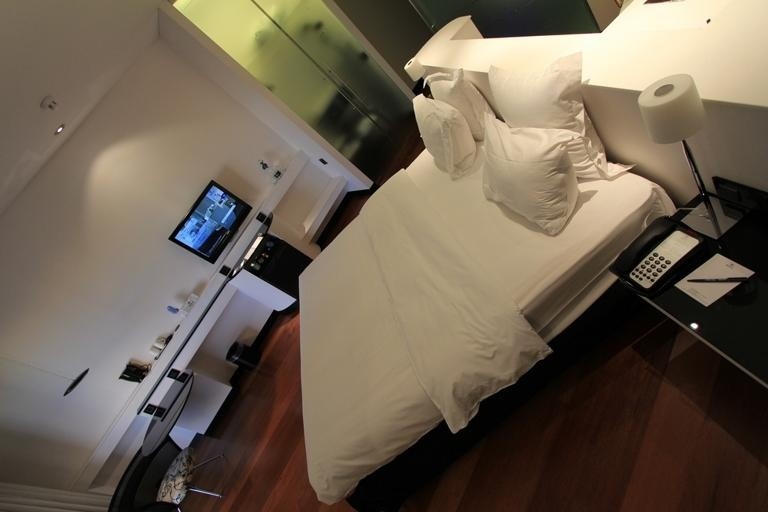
[607,175,768,387]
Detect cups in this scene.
[153,336,167,350]
[147,347,160,358]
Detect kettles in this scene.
[118,362,150,383]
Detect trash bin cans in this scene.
[226,341,258,370]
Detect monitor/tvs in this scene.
[168,180,252,265]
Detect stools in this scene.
[156,447,223,512]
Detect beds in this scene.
[298,165,676,511]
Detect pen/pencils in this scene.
[687,277,748,282]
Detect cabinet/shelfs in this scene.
[68,148,348,492]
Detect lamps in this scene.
[637,73,744,240]
[63,368,90,396]
[403,57,426,83]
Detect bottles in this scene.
[258,158,283,181]
[167,305,187,321]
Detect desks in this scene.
[142,369,194,457]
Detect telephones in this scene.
[612,216,705,293]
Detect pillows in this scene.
[412,93,477,181]
[482,111,579,237]
[422,67,496,141]
[487,49,637,180]
